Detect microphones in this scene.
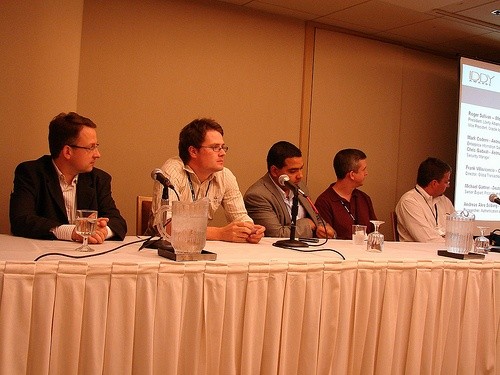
[278,174,308,198]
[489,194,500,205]
[151,168,174,189]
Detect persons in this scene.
[394,157,458,242]
[313,148,380,240]
[9,111,128,244]
[243,140,337,239]
[151,117,266,244]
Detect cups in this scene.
[351,225,367,246]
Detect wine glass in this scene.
[74,210,97,252]
[368,220,386,253]
[474,226,489,255]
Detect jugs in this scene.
[156,200,209,255]
[445,216,475,255]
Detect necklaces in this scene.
[414,185,438,226]
[185,170,213,202]
[339,195,360,225]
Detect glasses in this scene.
[68,144,100,151]
[199,144,228,152]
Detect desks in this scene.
[0,234,500,374]
